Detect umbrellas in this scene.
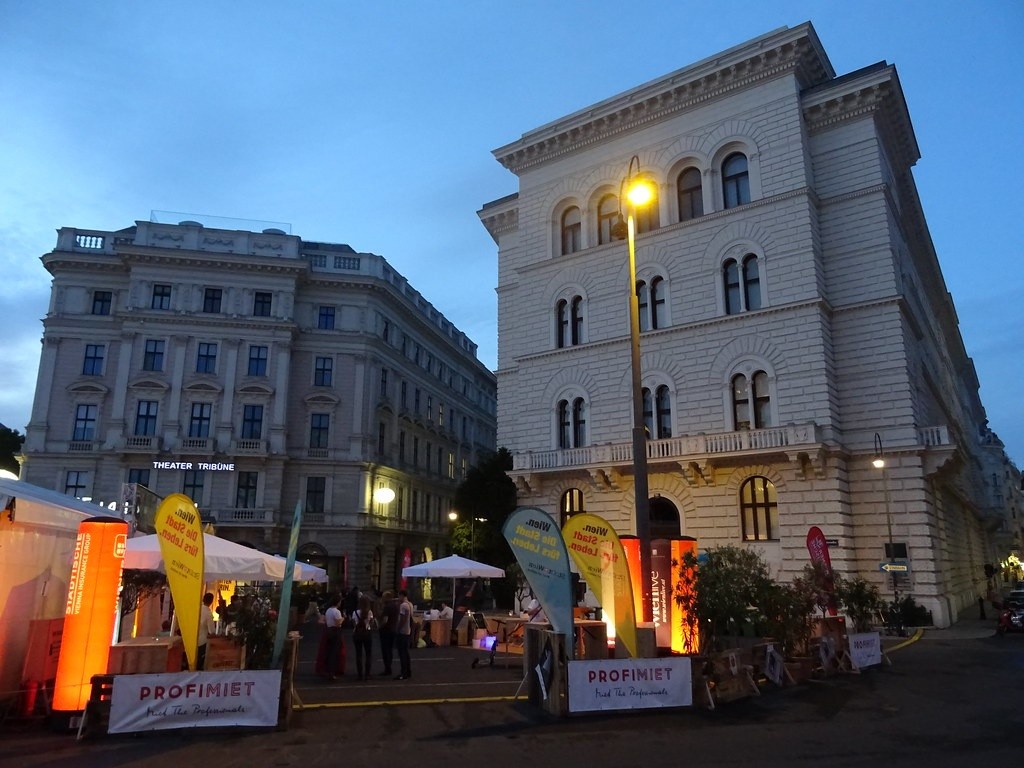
[122,530,328,636]
[402,554,506,613]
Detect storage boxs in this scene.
[473,629,486,639]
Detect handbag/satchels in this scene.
[410,616,413,627]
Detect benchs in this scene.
[86,673,113,740]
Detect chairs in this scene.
[450,611,464,645]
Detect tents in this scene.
[0,479,137,702]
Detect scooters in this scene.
[997,600,1024,634]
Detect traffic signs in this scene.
[879,561,910,575]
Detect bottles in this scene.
[215,619,231,635]
[585,603,591,619]
[468,608,471,617]
[303,602,321,622]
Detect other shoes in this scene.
[365,674,372,680]
[385,665,392,675]
[392,673,411,681]
[356,674,363,680]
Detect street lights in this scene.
[872,432,897,595]
[449,499,473,560]
[627,153,661,655]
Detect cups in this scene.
[577,601,587,619]
[150,632,160,644]
[508,610,514,618]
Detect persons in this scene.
[290,582,387,630]
[316,590,413,682]
[528,598,549,623]
[156,592,215,671]
[413,599,454,630]
[215,589,279,639]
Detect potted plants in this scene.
[238,600,296,731]
[676,548,885,707]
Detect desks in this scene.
[521,620,607,694]
[101,635,184,700]
[204,633,248,670]
[428,619,450,647]
[492,617,529,669]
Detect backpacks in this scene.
[353,611,370,644]
